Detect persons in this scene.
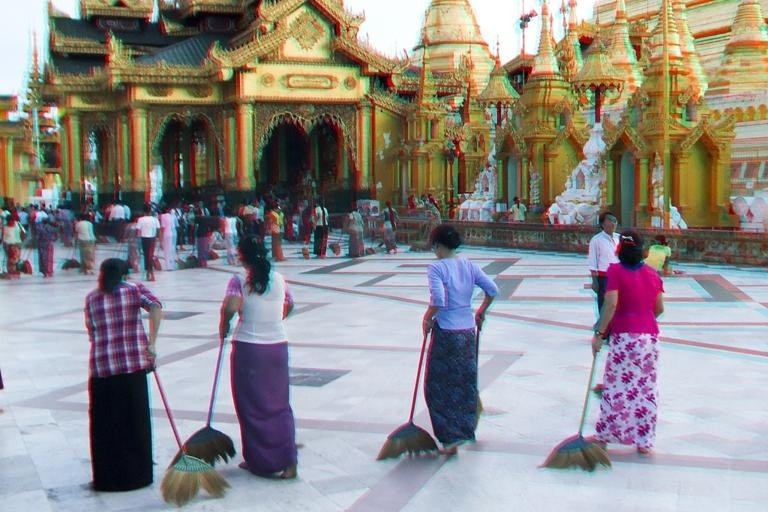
[222,198,269,265]
[54,200,161,280]
[1,203,54,280]
[267,197,314,262]
[422,221,499,456]
[312,200,328,258]
[505,196,527,221]
[83,257,164,492]
[346,207,364,258]
[585,229,663,463]
[407,194,440,219]
[381,200,399,254]
[643,232,676,278]
[587,211,624,316]
[158,201,226,271]
[217,233,299,480]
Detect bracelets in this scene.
[594,329,605,336]
